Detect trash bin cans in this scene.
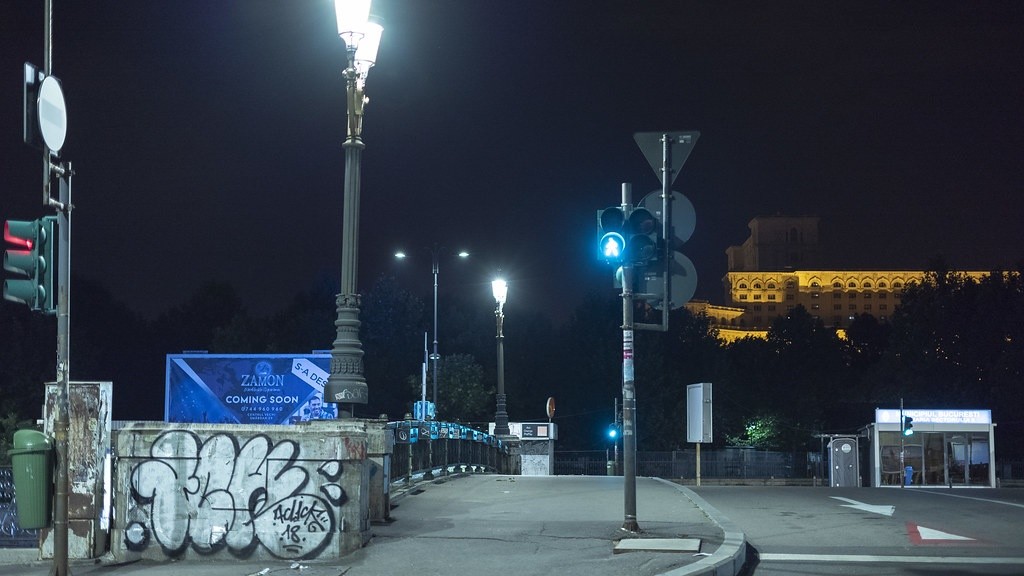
[831,438,857,486]
[607,461,616,476]
[6,428,56,532]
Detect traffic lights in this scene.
[0,219,46,314]
[595,207,628,265]
[627,207,660,263]
[609,426,620,440]
[905,416,913,434]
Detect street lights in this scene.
[323,1,385,403]
[490,266,509,435]
[394,249,470,421]
